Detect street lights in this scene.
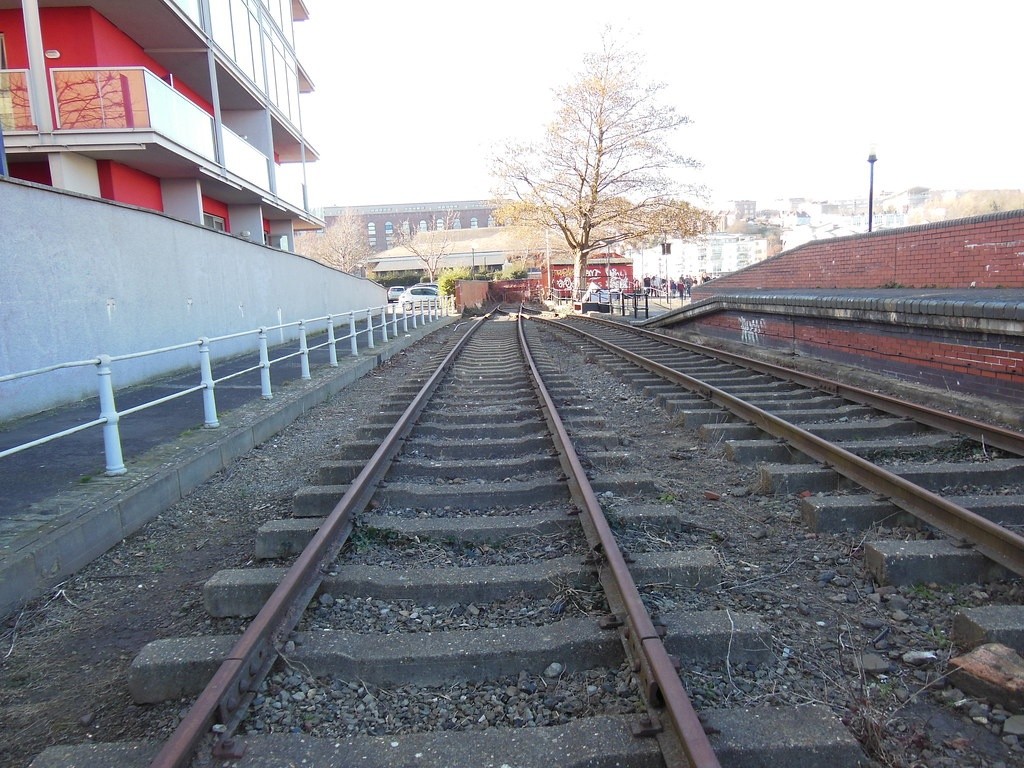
[865,150,877,233]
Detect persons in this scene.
[634,272,719,300]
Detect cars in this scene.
[387,285,406,302]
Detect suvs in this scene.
[400,282,450,311]
